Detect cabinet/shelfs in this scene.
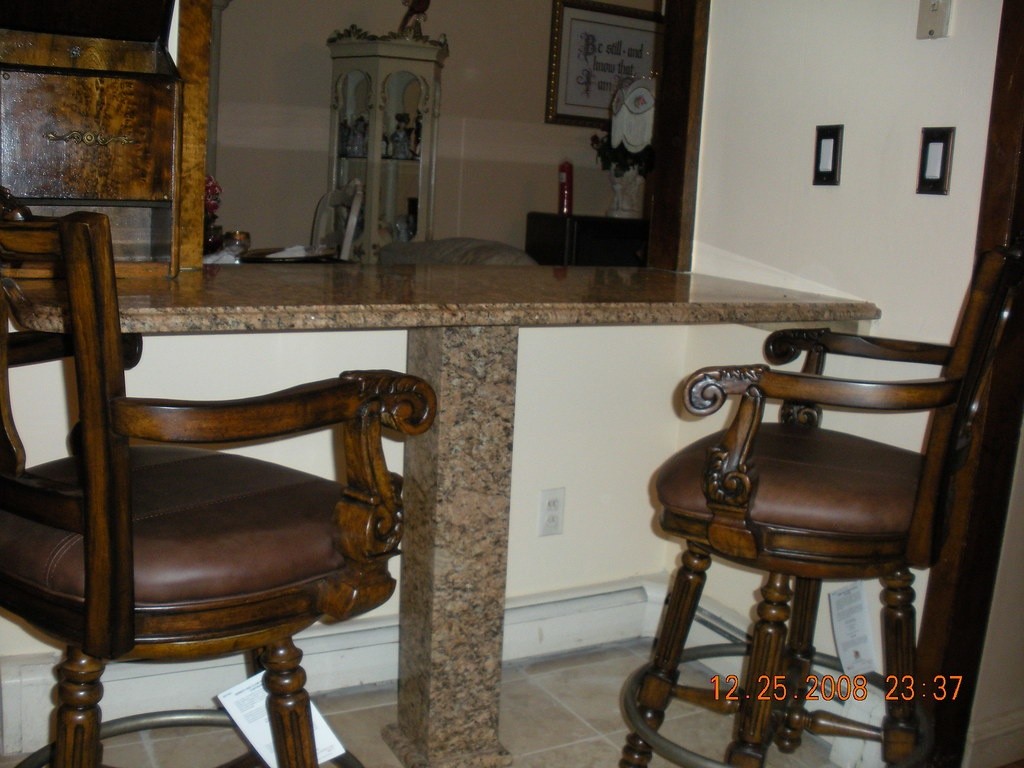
[327,25,452,251]
[0,29,184,283]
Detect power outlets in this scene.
[917,0,953,40]
[540,487,565,537]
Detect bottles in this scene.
[558,160,573,215]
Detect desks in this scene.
[0,263,879,768]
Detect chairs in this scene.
[311,177,361,259]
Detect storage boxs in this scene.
[524,211,656,266]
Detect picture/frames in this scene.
[545,0,656,133]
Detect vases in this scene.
[203,214,223,257]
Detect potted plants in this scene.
[589,129,653,220]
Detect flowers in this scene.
[206,172,224,217]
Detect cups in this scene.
[225,230,251,256]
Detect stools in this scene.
[2,185,435,768]
[618,242,1012,768]
